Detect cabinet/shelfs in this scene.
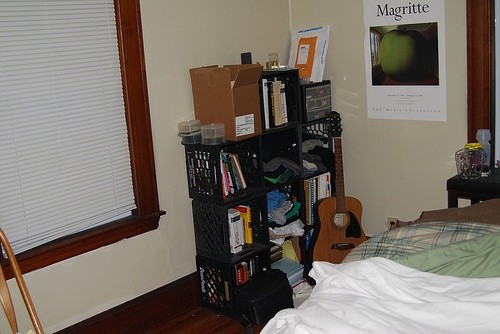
[178,114,334,321]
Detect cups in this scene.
[268,52,281,70]
[455,148,486,180]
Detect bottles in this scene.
[476,129,491,178]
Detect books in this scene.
[187,78,331,304]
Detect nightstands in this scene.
[447,168,500,208]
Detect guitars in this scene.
[305,111,367,286]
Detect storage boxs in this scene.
[189,63,263,141]
[300,79,332,123]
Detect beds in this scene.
[259,199,500,334]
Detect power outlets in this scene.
[388,217,398,229]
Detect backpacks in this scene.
[236,267,294,334]
